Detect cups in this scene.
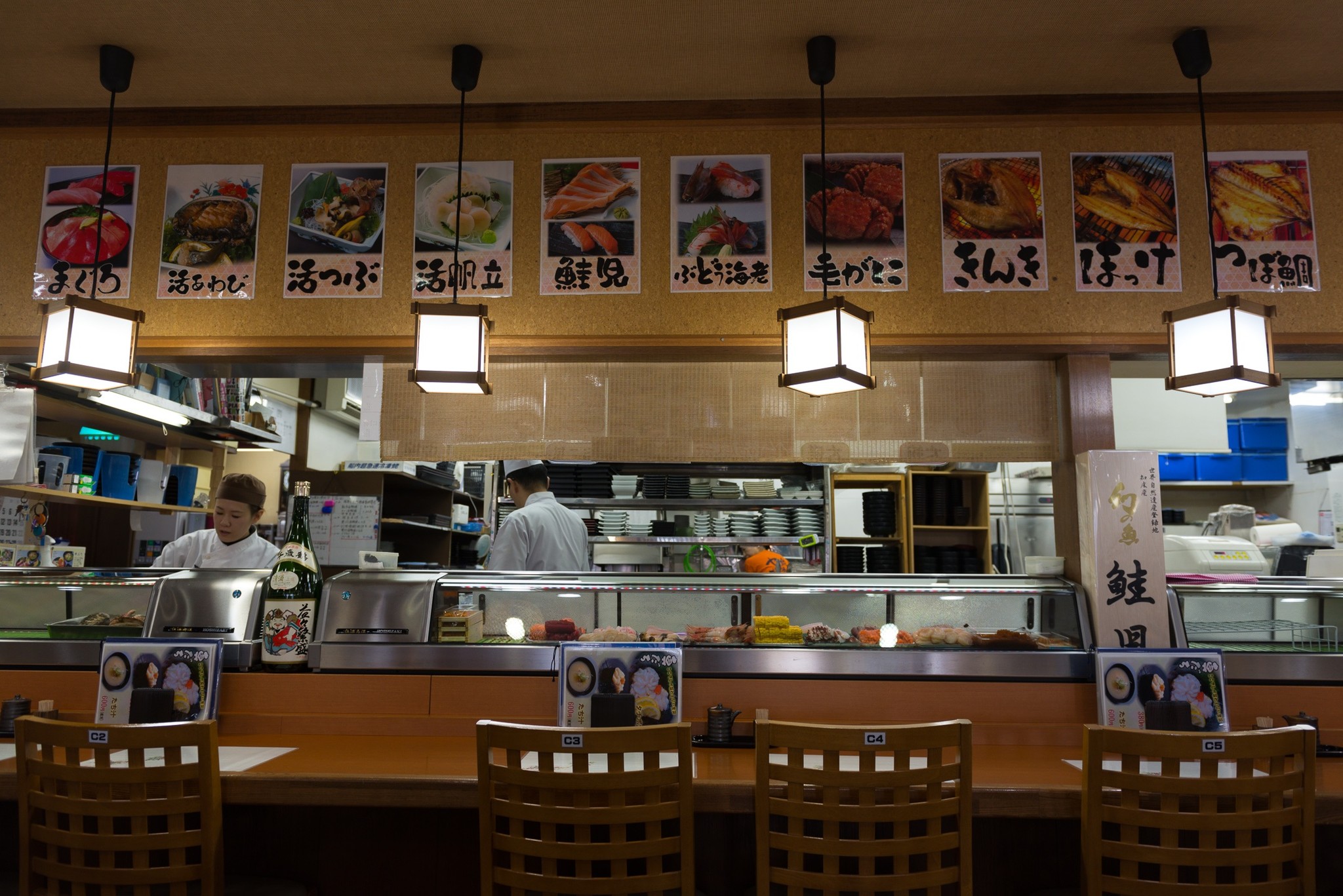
[32,709,59,720]
[1252,724,1268,730]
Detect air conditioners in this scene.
[313,376,362,429]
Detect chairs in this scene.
[752,717,975,895]
[1081,723,1319,896]
[475,718,698,896]
[14,714,224,896]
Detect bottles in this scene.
[259,481,323,673]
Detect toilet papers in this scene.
[1249,522,1303,546]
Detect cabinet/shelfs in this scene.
[1,363,284,516]
[489,459,830,573]
[832,469,994,576]
[286,467,485,569]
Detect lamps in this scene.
[406,42,495,397]
[78,386,193,429]
[1160,27,1282,398]
[30,43,146,391]
[777,35,878,400]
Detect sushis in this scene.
[561,222,618,256]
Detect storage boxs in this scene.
[135,372,171,397]
[245,409,268,430]
[344,441,486,532]
[1158,417,1289,482]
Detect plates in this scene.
[675,158,766,223]
[838,476,984,573]
[171,195,255,244]
[39,442,140,485]
[542,168,638,222]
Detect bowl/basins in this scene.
[566,656,596,697]
[101,651,131,692]
[42,206,131,267]
[415,166,511,251]
[289,171,384,254]
[1104,663,1136,702]
[499,465,825,537]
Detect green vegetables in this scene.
[162,218,255,263]
[297,171,380,237]
[66,202,99,217]
[683,207,729,257]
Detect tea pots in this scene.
[1283,711,1320,749]
[0,694,31,732]
[707,702,742,741]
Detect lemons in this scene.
[635,698,661,720]
[1191,706,1205,727]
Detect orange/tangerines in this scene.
[173,691,190,714]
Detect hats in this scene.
[503,459,543,477]
[214,473,267,509]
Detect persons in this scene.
[487,461,593,572]
[149,473,283,568]
[738,546,790,574]
